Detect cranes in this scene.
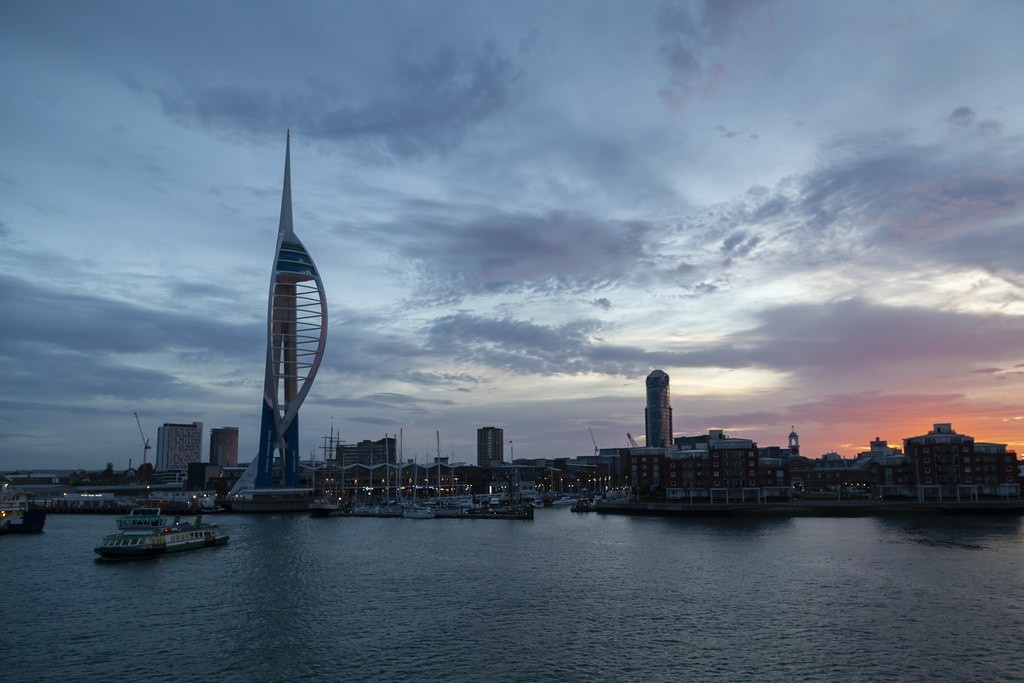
[627,432,639,448]
[132,412,151,481]
[589,427,599,456]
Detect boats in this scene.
[0,507,46,534]
[94,507,230,560]
[552,496,578,506]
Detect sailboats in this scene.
[308,415,542,518]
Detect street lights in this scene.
[145,484,150,506]
